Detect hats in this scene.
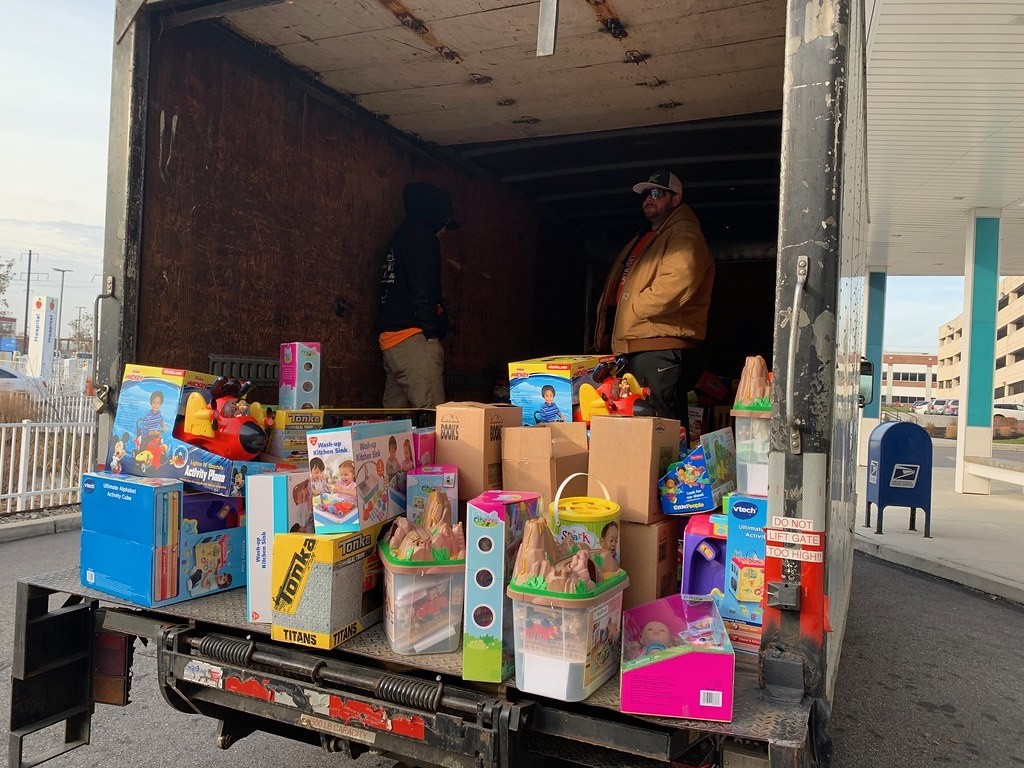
[446,210,462,230]
[632,170,682,193]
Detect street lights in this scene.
[51,268,72,394]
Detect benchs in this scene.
[963,456,1024,485]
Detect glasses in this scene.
[642,189,674,200]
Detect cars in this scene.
[909,399,958,416]
[0,368,47,402]
[994,403,1024,421]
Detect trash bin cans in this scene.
[862,421,933,538]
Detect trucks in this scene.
[0,0,876,768]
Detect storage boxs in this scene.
[79,341,774,722]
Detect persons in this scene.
[386,436,414,482]
[692,420,701,435]
[139,391,165,452]
[599,521,618,559]
[375,188,462,407]
[234,400,248,417]
[310,457,357,495]
[593,170,715,449]
[188,568,232,597]
[293,478,312,509]
[540,385,566,423]
[619,380,632,399]
[631,621,671,659]
